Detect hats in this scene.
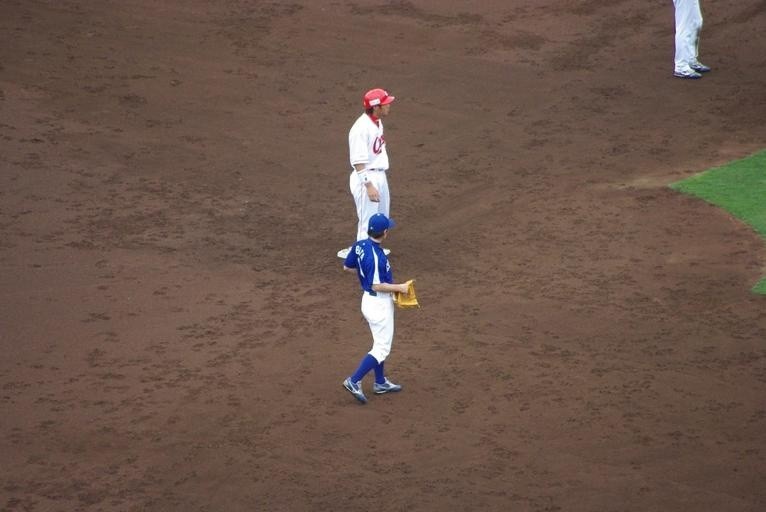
[368,214,392,231]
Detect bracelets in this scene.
[357,170,370,185]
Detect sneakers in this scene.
[692,63,710,72]
[673,67,700,79]
[342,377,367,403]
[373,378,402,395]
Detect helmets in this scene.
[364,90,394,109]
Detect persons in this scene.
[672,0,712,80]
[343,213,410,404]
[338,89,396,259]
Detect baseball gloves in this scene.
[393,279,420,308]
